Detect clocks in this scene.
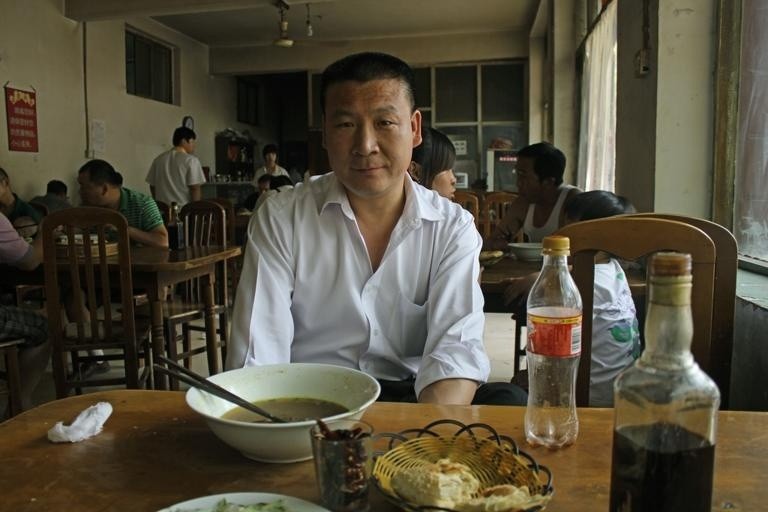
[183,116,194,131]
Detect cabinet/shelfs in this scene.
[203,182,260,212]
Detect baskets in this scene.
[369,419,555,512]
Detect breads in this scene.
[391,458,548,511]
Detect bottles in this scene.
[524,233,582,448]
[612,253,716,510]
[50,225,119,262]
[168,201,185,251]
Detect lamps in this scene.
[305,5,315,36]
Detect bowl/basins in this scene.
[185,364,380,463]
[507,241,545,262]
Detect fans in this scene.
[274,4,297,49]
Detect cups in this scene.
[309,419,376,510]
[202,167,209,183]
[237,168,241,182]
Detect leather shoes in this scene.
[73,360,111,378]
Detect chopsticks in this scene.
[151,353,285,423]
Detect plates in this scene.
[158,491,333,512]
[479,250,509,266]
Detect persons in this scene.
[226,51,529,404]
[0,166,40,225]
[31,181,76,221]
[500,190,642,407]
[237,174,272,222]
[488,142,585,250]
[144,127,207,217]
[407,127,457,203]
[251,144,290,190]
[63,160,171,380]
[0,213,58,418]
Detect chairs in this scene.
[162,201,229,390]
[572,212,740,406]
[42,207,153,397]
[454,190,478,233]
[154,202,172,231]
[17,285,59,332]
[483,190,523,242]
[0,338,25,421]
[542,218,716,405]
[212,198,238,300]
